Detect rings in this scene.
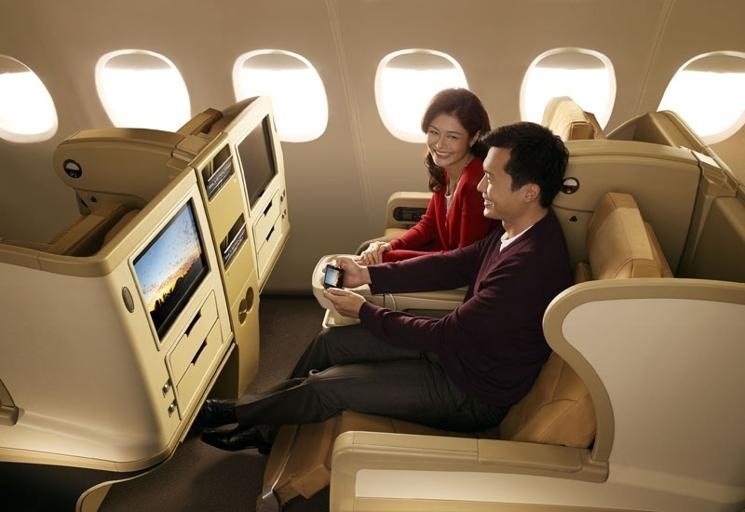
[382,245,387,250]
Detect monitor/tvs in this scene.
[133,198,209,342]
[238,117,275,208]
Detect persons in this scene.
[190,121,577,456]
[354,84,500,268]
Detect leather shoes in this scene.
[200,421,273,454]
[196,399,239,429]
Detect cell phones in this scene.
[324,264,345,289]
